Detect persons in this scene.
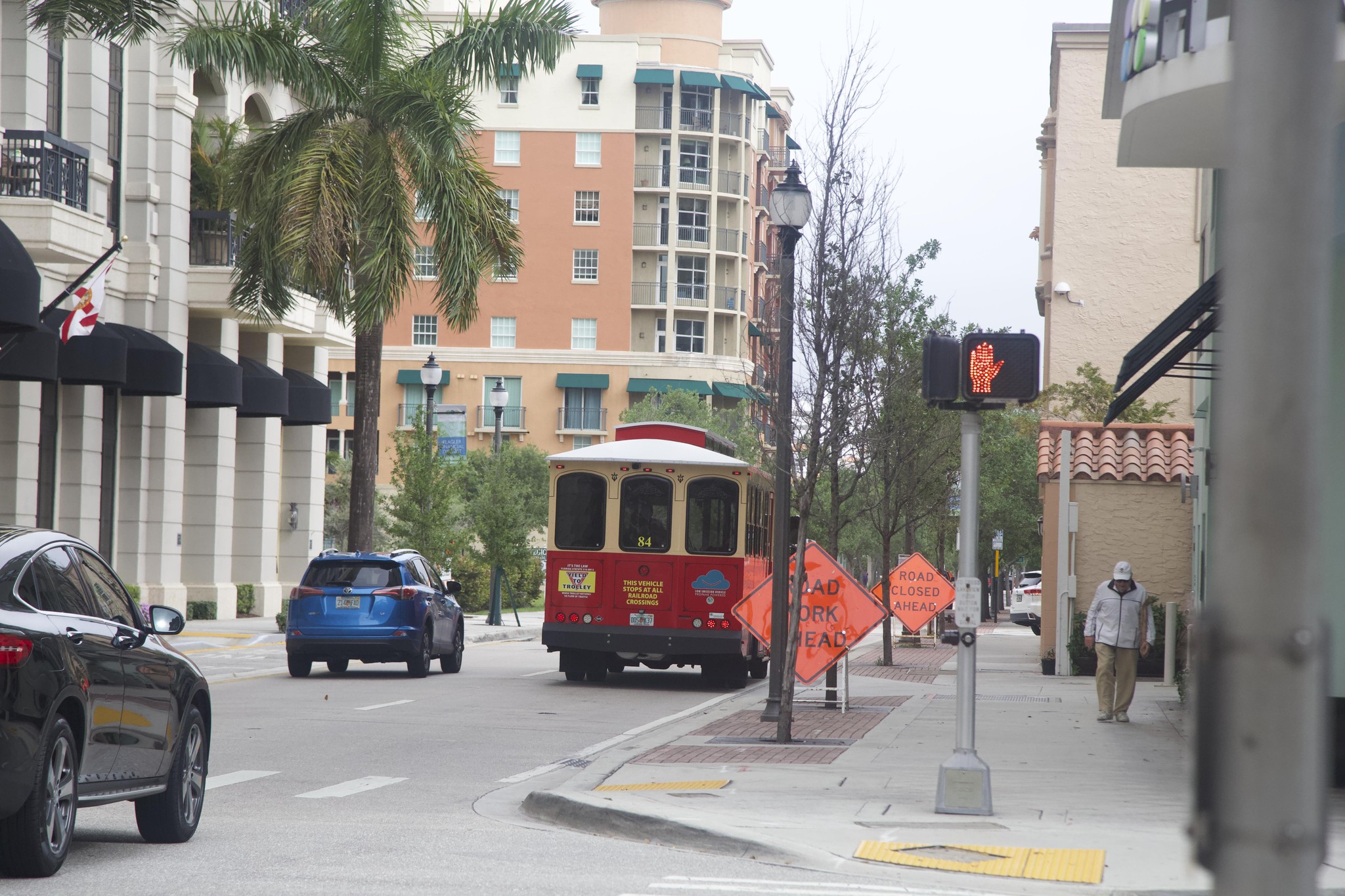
[1083,560,1155,722]
[862,570,869,589]
[645,503,665,530]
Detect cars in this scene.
[934,568,956,609]
[0,524,212,879]
[986,565,1015,595]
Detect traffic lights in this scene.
[961,332,1041,403]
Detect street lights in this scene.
[754,158,816,724]
[483,379,509,623]
[420,351,443,560]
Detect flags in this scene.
[59,252,120,342]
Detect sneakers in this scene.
[1096,710,1114,720]
[1116,711,1130,722]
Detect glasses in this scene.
[1116,580,1130,583]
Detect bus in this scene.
[540,420,803,691]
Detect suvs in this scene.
[1009,570,1042,635]
[284,549,465,678]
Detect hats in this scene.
[1113,561,1132,580]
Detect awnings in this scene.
[0,217,41,329]
[281,368,332,426]
[43,306,127,385]
[103,322,183,397]
[236,355,289,418]
[186,342,243,409]
[0,322,58,381]
